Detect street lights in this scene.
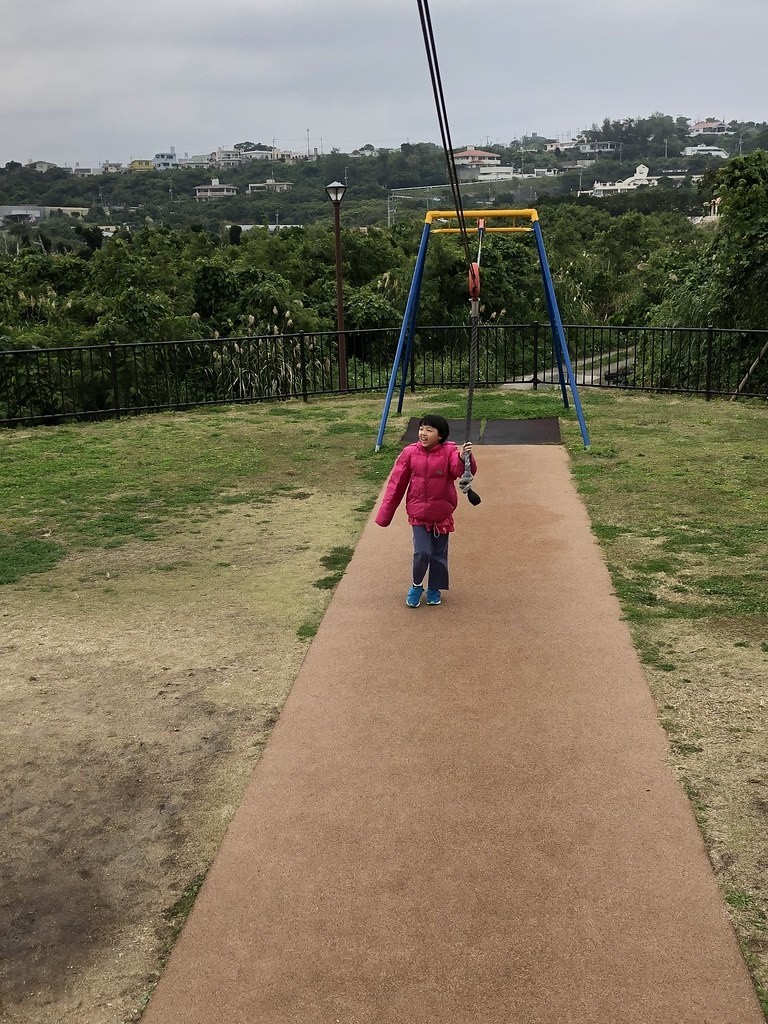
[324,180,347,391]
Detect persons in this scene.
[375,415,477,607]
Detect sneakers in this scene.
[426,588,441,604]
[406,586,423,607]
